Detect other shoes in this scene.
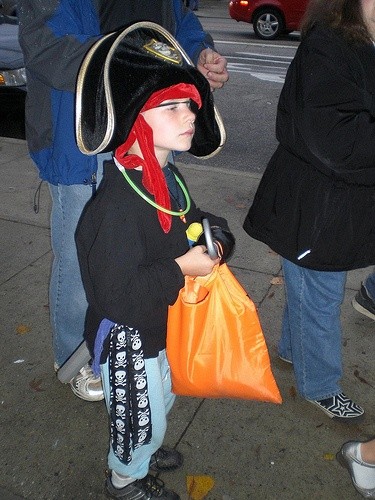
[337,440,375,497]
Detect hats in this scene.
[76,21,227,158]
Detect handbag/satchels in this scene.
[167,261,283,405]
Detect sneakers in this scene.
[149,448,182,470]
[307,393,366,423]
[54,362,104,402]
[352,286,375,320]
[105,471,181,500]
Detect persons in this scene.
[72,26,236,500]
[16,0,230,403]
[336,438,375,499]
[243,1,374,421]
[350,269,375,320]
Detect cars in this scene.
[0,13,28,109]
[228,0,313,40]
